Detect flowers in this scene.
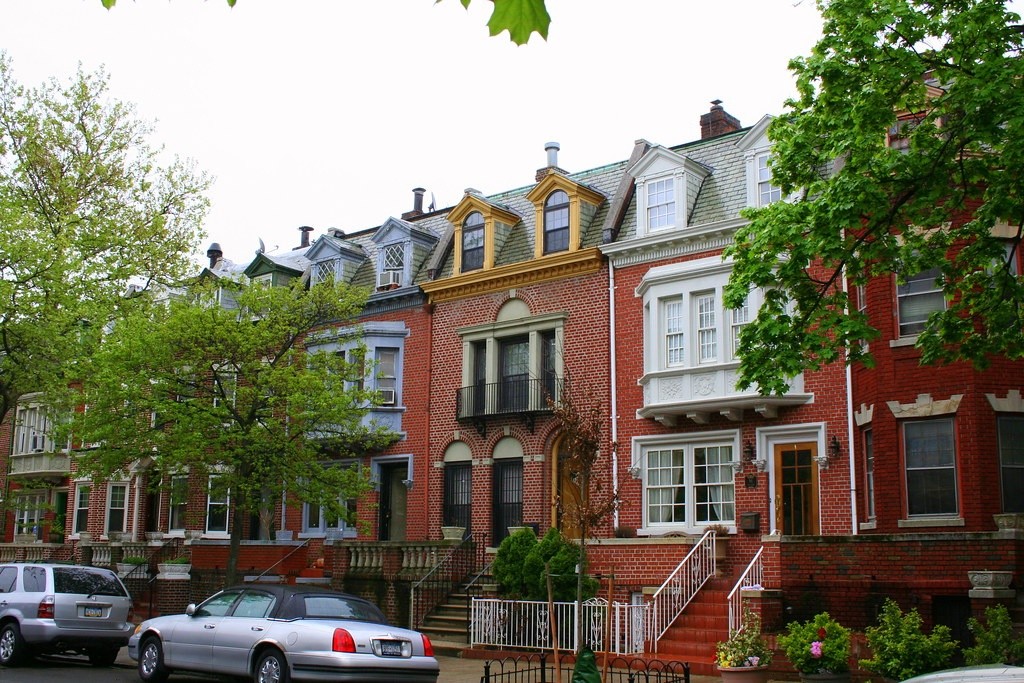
[775,610,852,675]
[712,597,773,667]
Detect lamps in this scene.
[743,440,753,461]
[829,435,841,458]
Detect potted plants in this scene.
[156,557,191,580]
[116,557,149,578]
[48,517,65,543]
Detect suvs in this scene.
[0,562,138,670]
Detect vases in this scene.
[799,671,851,683]
[716,661,772,683]
[14,534,38,543]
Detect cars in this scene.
[128,582,442,683]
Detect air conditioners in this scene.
[375,389,394,404]
[377,271,403,286]
